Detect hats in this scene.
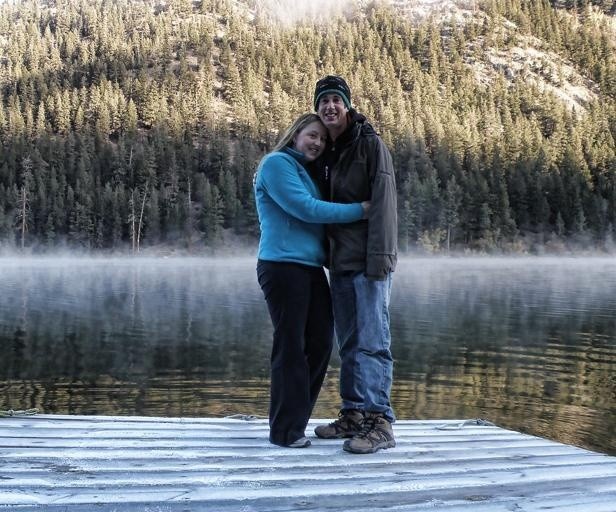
[314,75,351,111]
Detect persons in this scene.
[253,113,370,450]
[252,75,399,456]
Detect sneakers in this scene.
[315,409,366,439]
[343,411,396,454]
[288,436,311,448]
